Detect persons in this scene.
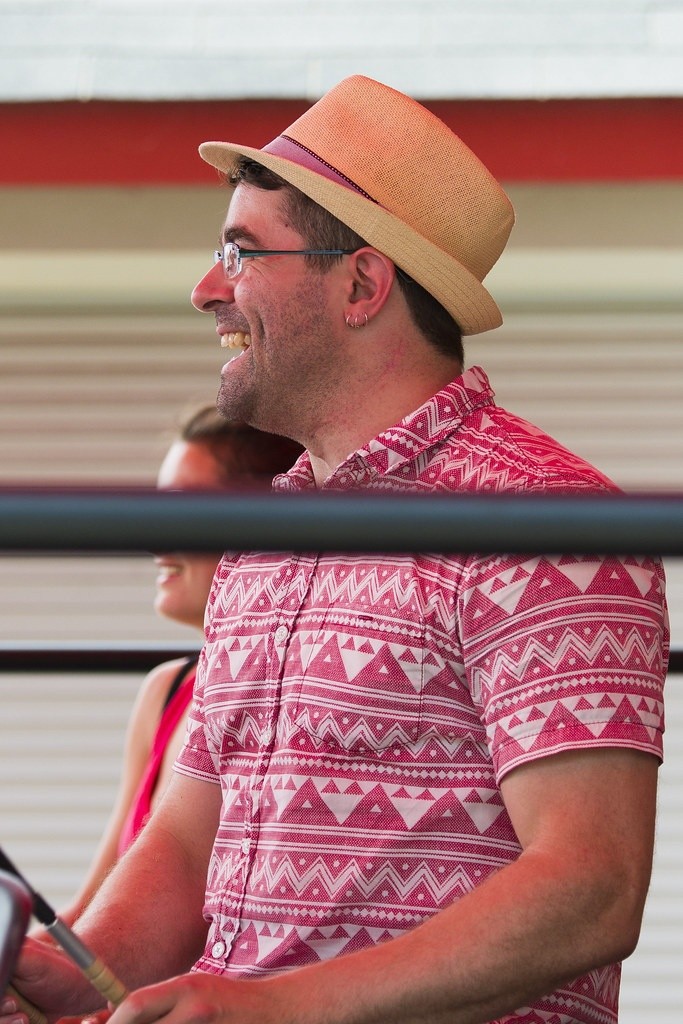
[0,405,305,1024]
[0,71,669,1024]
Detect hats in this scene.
[197,74,516,336]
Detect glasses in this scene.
[212,242,414,286]
